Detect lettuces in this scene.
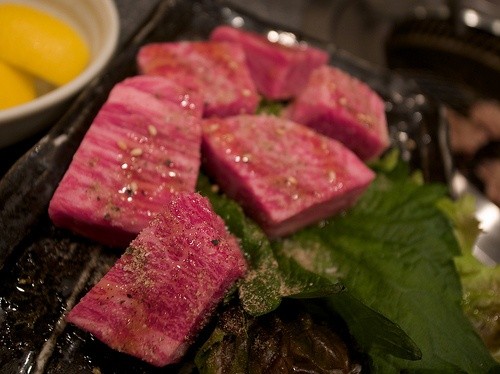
[190,95,500,374]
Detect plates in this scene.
[0,1,493,374]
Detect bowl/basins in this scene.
[0,0,120,122]
